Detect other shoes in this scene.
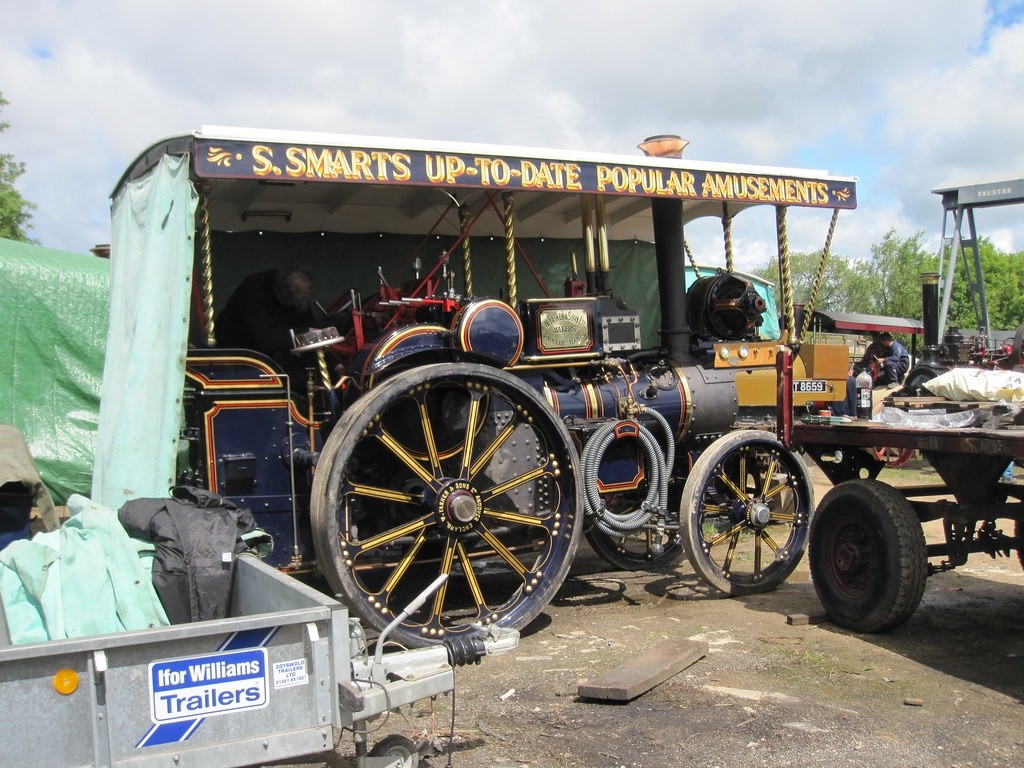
[888,382,898,389]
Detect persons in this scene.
[877,332,909,388]
[860,332,892,370]
[216,269,346,393]
[1003,462,1017,484]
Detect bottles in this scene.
[856,367,873,420]
